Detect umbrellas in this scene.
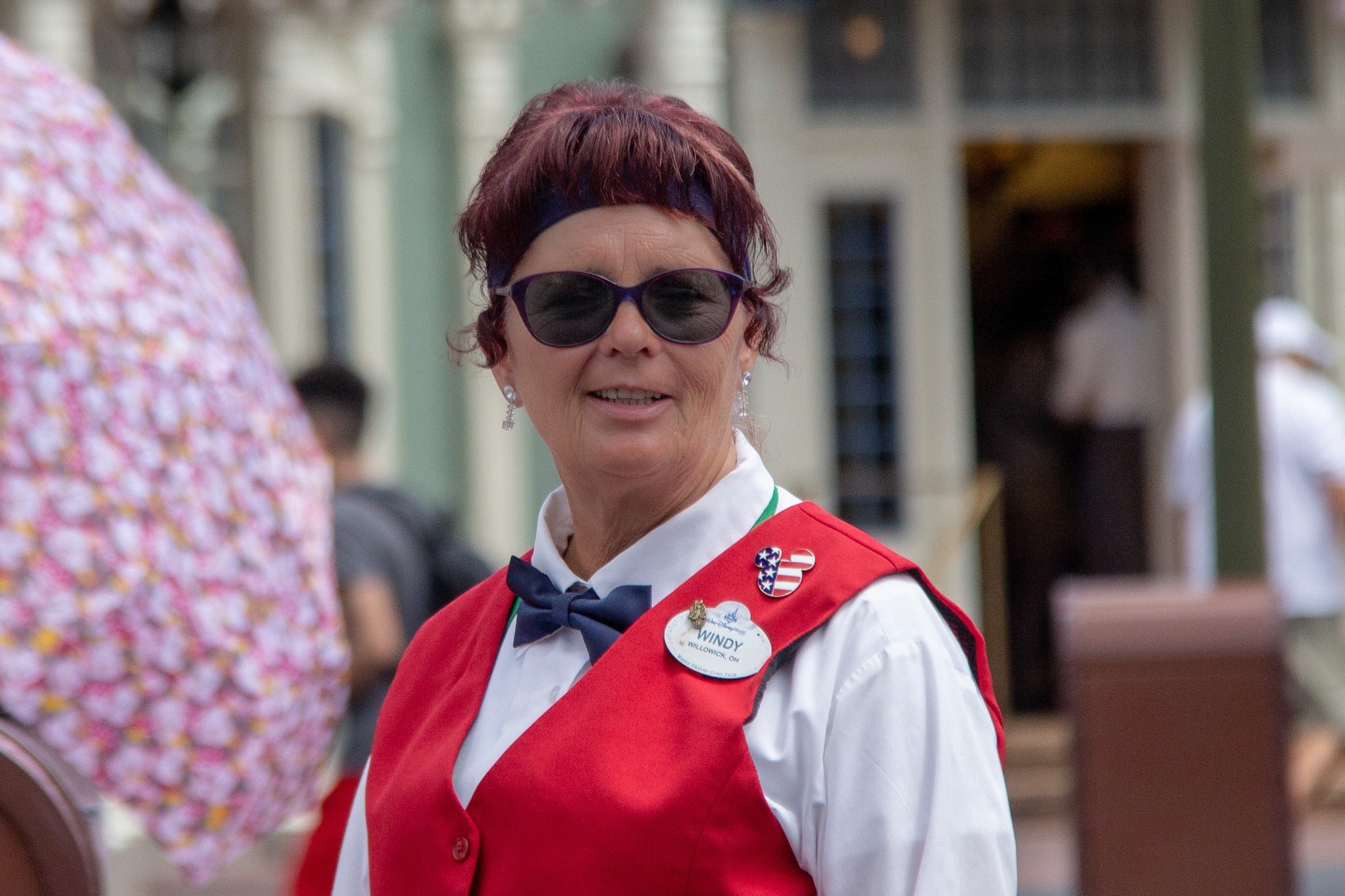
[0,38,349,896]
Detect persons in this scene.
[1038,230,1157,700]
[294,363,432,773]
[0,708,108,896]
[1163,300,1345,816]
[328,86,1020,896]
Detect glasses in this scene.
[494,267,758,350]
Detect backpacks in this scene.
[342,483,493,609]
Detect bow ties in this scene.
[506,555,651,667]
